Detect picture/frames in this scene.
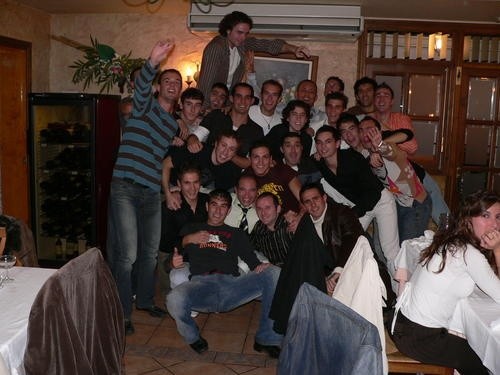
[253,52,320,106]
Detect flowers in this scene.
[68,33,143,95]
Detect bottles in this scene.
[56,239,96,259]
[436,212,452,234]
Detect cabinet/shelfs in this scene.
[25,91,123,269]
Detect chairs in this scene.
[275,234,454,375]
[0,214,126,375]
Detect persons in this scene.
[393,190,500,375]
[106,11,450,357]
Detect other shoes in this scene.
[254,342,281,359]
[191,338,208,352]
[136,303,166,316]
[124,320,134,335]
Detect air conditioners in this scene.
[187,3,365,44]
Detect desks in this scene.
[394,235,500,375]
[0,266,58,375]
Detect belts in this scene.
[202,272,226,275]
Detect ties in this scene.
[237,204,251,233]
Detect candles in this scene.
[187,69,191,82]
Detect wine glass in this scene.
[0,255,17,283]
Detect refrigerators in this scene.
[28,91,121,271]
